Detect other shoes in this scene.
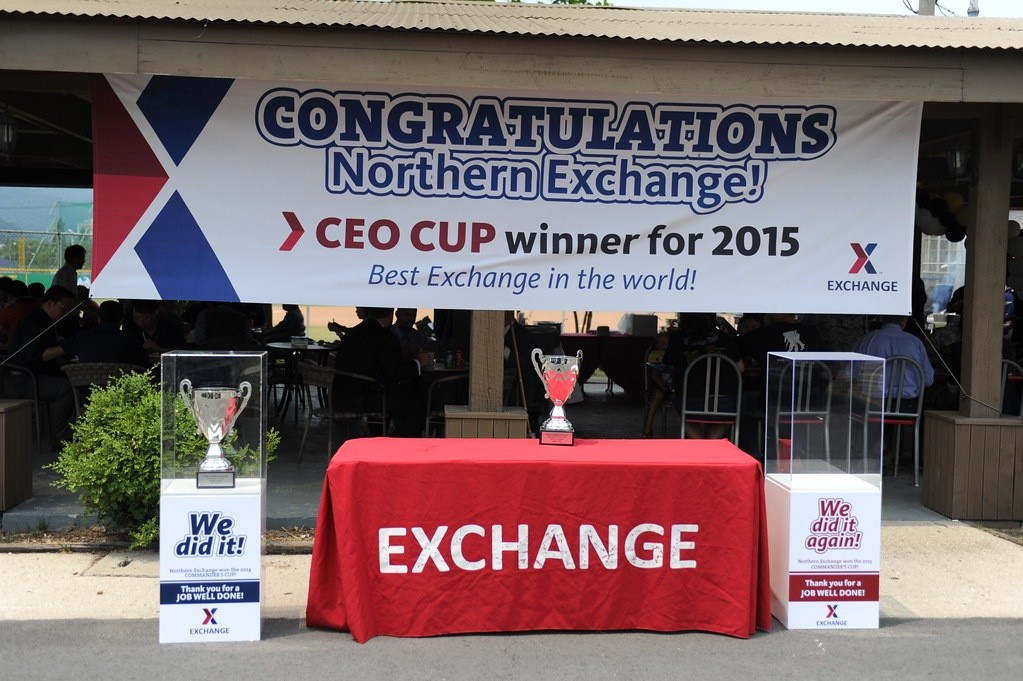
[52,443,63,451]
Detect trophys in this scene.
[180,379,252,489]
[531,348,584,446]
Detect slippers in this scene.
[642,428,653,439]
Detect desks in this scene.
[304,437,771,644]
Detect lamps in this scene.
[947,132,979,188]
[0,102,18,161]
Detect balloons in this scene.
[916,177,1023,303]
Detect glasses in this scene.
[52,300,71,313]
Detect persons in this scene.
[0,245,542,453]
[640,277,964,471]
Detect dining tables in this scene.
[558,332,659,395]
[419,361,468,437]
[266,342,339,421]
[250,327,272,352]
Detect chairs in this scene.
[0,308,1023,494]
